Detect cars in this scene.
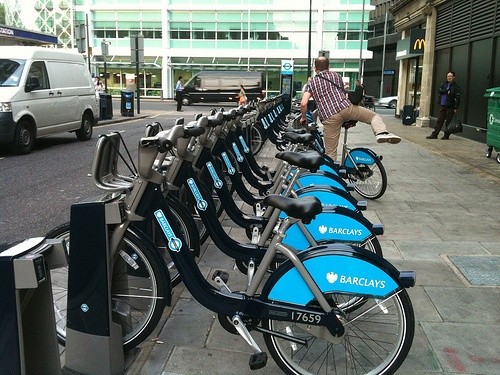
[378,95,398,109]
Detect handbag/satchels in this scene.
[244,95,247,100]
[450,111,464,133]
[239,95,245,102]
[348,91,359,105]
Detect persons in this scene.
[425,71,460,140]
[301,76,317,125]
[300,56,401,162]
[94,76,104,97]
[355,80,363,105]
[236,85,247,107]
[360,84,366,96]
[343,82,350,99]
[176,76,184,112]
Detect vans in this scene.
[174,71,263,104]
[0,46,99,155]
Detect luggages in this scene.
[403,96,416,125]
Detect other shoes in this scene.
[441,134,450,140]
[376,133,401,144]
[426,135,438,139]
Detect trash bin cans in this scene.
[99,94,113,119]
[121,90,134,117]
[484,86,500,163]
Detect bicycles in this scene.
[42,125,417,375]
[136,92,389,316]
